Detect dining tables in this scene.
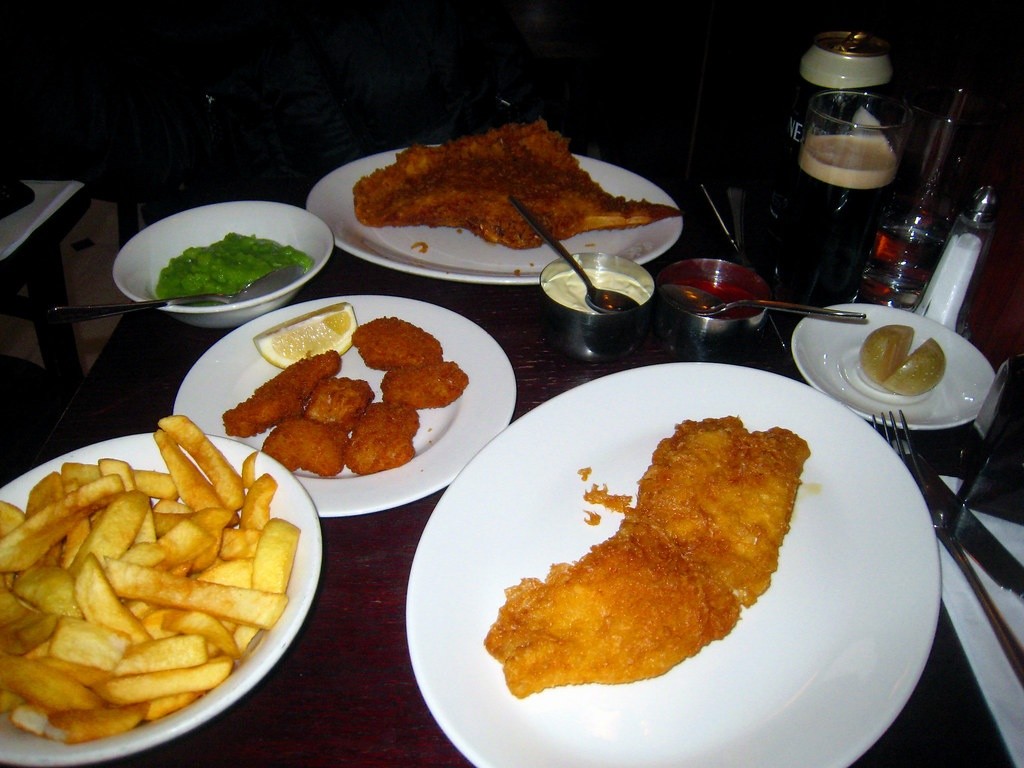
[0,179,1024,768]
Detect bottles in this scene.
[909,186,998,341]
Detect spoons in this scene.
[661,283,868,320]
[508,195,641,313]
[47,266,305,326]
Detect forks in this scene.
[869,411,1024,692]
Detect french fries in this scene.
[0,414,301,744]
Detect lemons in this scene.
[253,301,358,369]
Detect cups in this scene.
[866,86,1009,291]
[784,91,913,301]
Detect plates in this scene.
[0,432,322,766]
[171,295,515,517]
[405,362,940,768]
[307,144,684,284]
[790,303,998,431]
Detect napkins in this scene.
[925,475,1024,768]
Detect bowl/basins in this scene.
[658,257,772,361]
[539,251,656,363]
[112,201,334,329]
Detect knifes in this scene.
[890,438,1023,600]
